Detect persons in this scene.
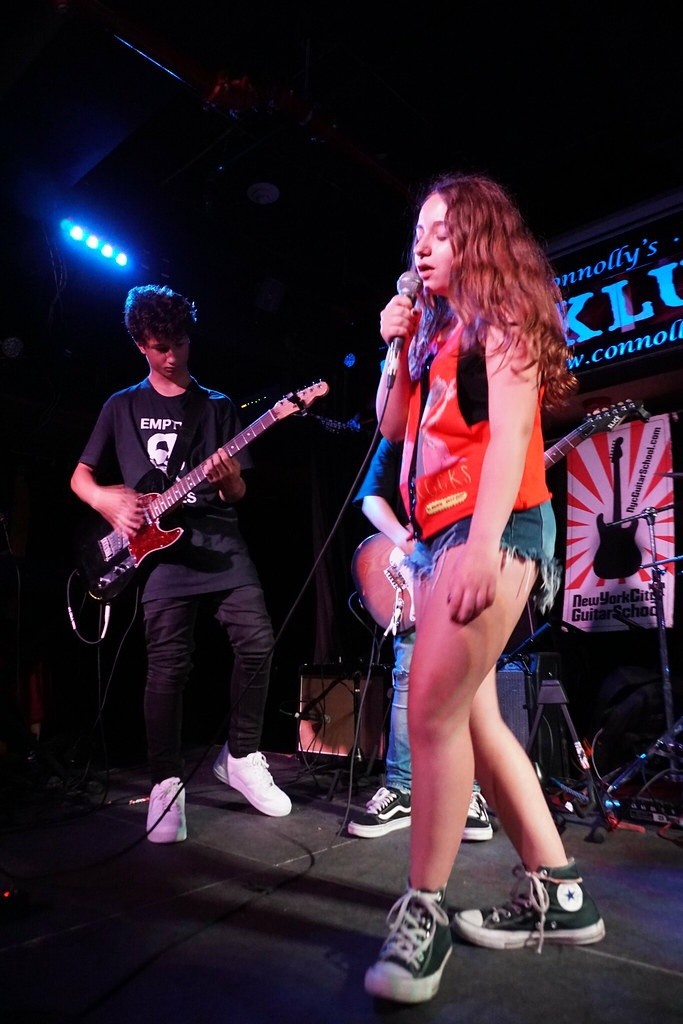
[71,284,293,841]
[346,440,494,840]
[367,177,605,1003]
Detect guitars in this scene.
[348,393,647,638]
[75,377,330,604]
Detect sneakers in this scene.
[212,741,293,817]
[454,853,606,954]
[364,875,453,1003]
[464,789,494,841]
[349,786,417,838]
[145,776,186,842]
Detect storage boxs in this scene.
[296,666,388,764]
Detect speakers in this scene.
[297,664,393,766]
[494,652,537,756]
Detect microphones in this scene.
[291,712,331,724]
[386,270,423,389]
